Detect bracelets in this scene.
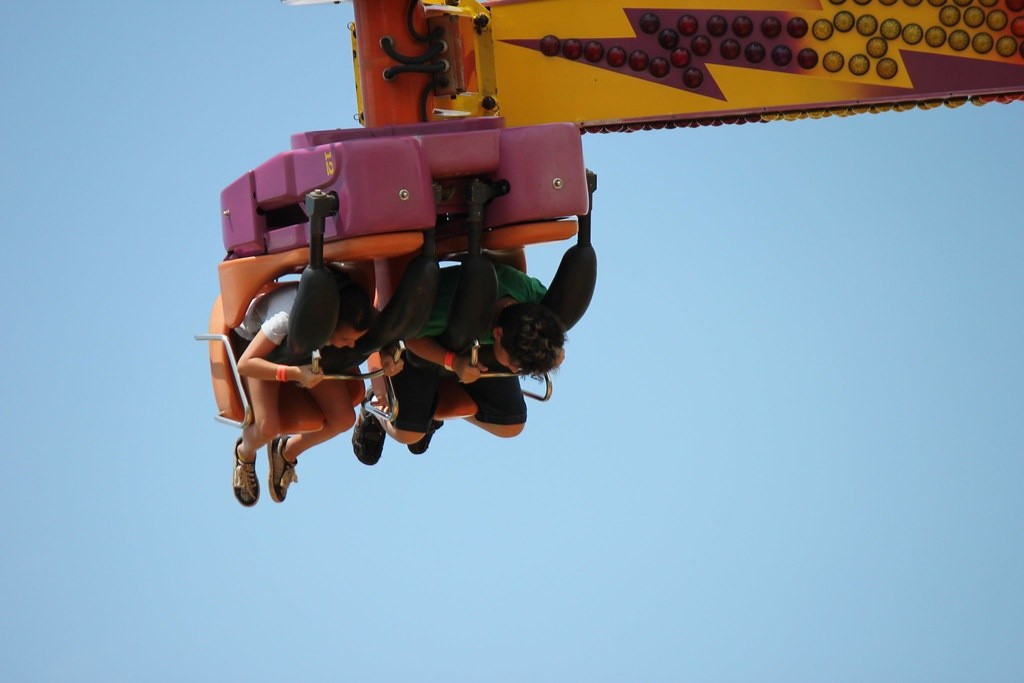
[444,352,456,371]
[275,365,286,381]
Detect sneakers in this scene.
[408,418,447,454]
[232,437,261,506]
[267,434,299,502]
[351,384,386,465]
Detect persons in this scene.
[351,256,567,465]
[228,281,405,509]
